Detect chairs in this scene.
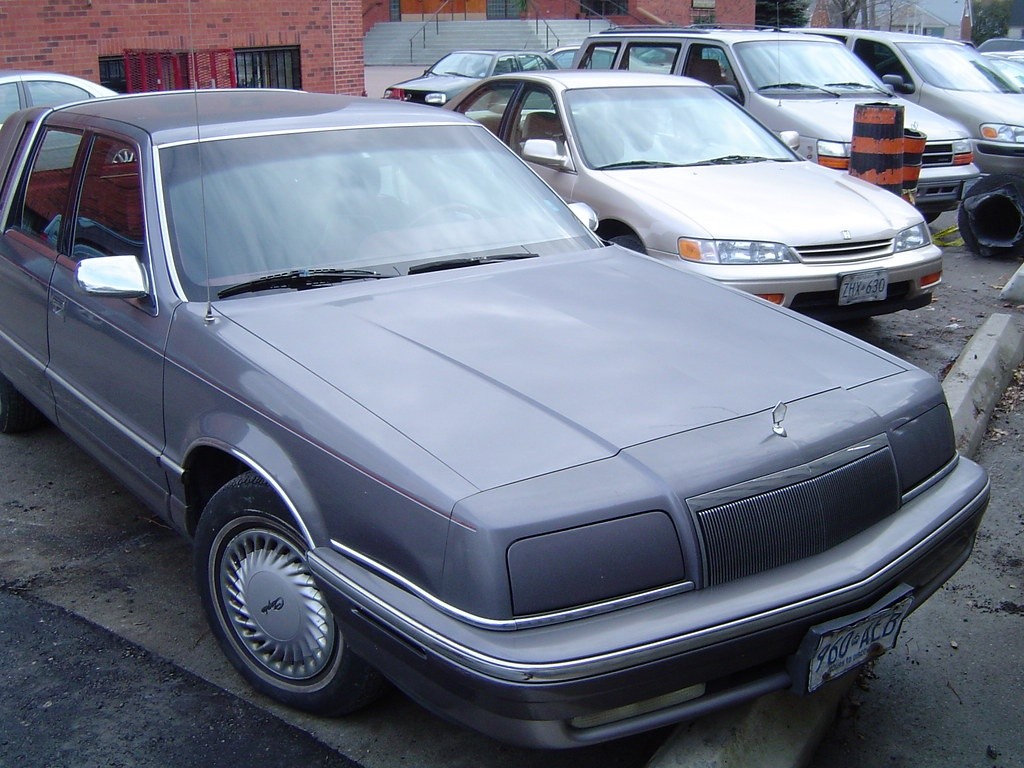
[689,59,722,85]
[522,112,566,144]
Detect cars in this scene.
[382,50,563,110]
[0,68,139,172]
[0,88,991,752]
[763,27,1024,187]
[519,44,672,72]
[442,69,946,320]
[977,37,1024,91]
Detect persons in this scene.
[712,21,723,29]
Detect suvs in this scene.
[568,24,978,225]
